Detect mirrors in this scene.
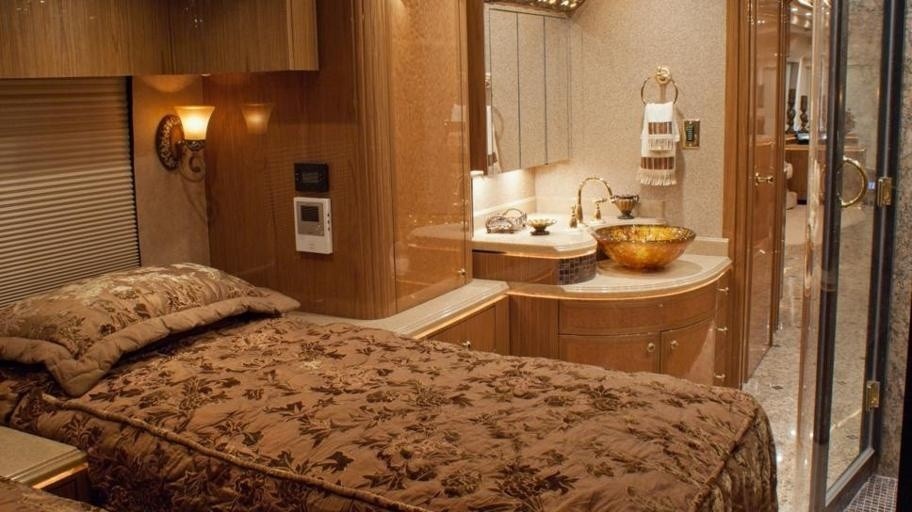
[484,7,571,173]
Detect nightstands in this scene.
[0,426,90,505]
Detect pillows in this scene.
[0,262,300,396]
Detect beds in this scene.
[0,311,779,512]
[0,475,107,512]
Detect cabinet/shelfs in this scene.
[716,263,737,389]
[557,281,718,389]
[427,304,496,354]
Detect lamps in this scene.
[155,106,215,182]
[239,103,273,152]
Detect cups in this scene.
[610,192,641,219]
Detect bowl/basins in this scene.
[591,222,697,273]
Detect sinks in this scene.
[589,223,698,274]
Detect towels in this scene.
[635,102,681,186]
[485,106,502,177]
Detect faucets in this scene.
[575,176,613,223]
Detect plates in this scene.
[524,217,558,236]
